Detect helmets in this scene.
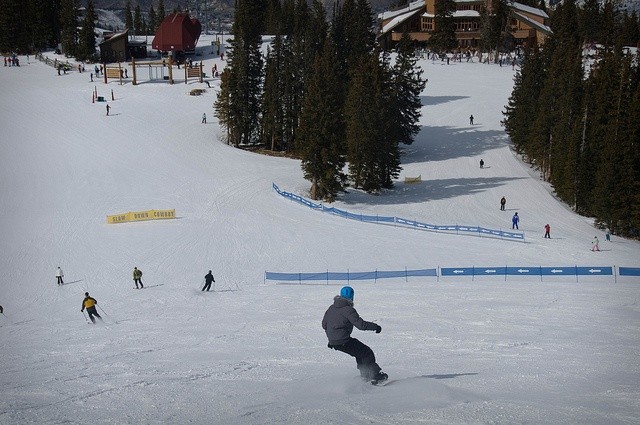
[341,287,354,299]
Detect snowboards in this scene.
[370,374,389,386]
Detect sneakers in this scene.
[374,372,388,380]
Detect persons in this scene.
[175,52,225,78]
[500,196,507,211]
[605,227,610,241]
[591,236,600,251]
[544,224,552,239]
[55,266,65,285]
[94,65,103,78]
[78,64,85,73]
[479,159,485,168]
[133,267,143,290]
[81,292,103,324]
[204,81,211,88]
[512,212,519,229]
[416,47,517,71]
[106,104,111,116]
[121,68,129,79]
[322,286,392,384]
[201,113,207,123]
[4,52,32,67]
[469,115,474,126]
[202,270,216,292]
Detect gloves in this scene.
[376,326,381,333]
[328,343,332,349]
